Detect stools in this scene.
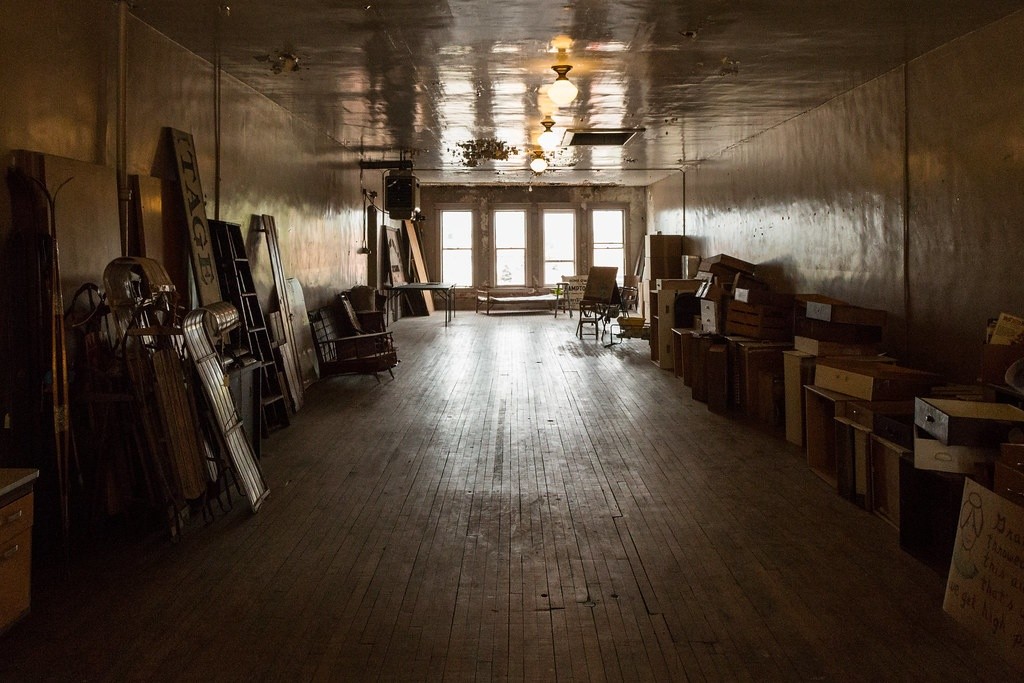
[554,282,573,317]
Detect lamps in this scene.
[547,65,578,106]
[538,117,558,150]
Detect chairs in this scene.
[579,300,599,340]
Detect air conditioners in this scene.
[384,169,421,222]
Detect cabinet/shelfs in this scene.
[319,332,398,382]
[0,488,31,635]
[649,254,1024,594]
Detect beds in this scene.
[476,289,566,315]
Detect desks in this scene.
[384,280,456,327]
